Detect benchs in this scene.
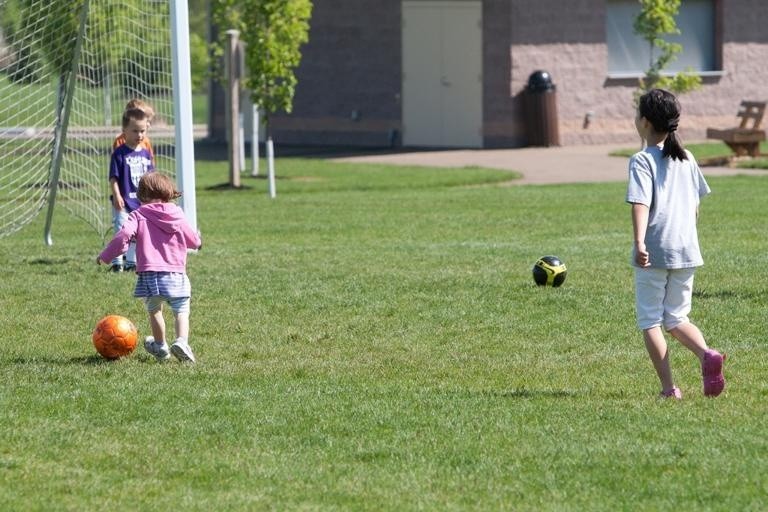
[704,99,767,161]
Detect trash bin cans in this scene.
[527,71,561,148]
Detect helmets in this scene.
[529,72,551,89]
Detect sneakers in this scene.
[661,350,726,398]
[171,338,195,362]
[145,336,169,359]
[113,265,135,272]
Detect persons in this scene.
[96,170,202,364]
[111,108,155,273]
[626,89,724,400]
[113,99,155,149]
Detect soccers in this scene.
[533,256,566,287]
[93,316,137,358]
